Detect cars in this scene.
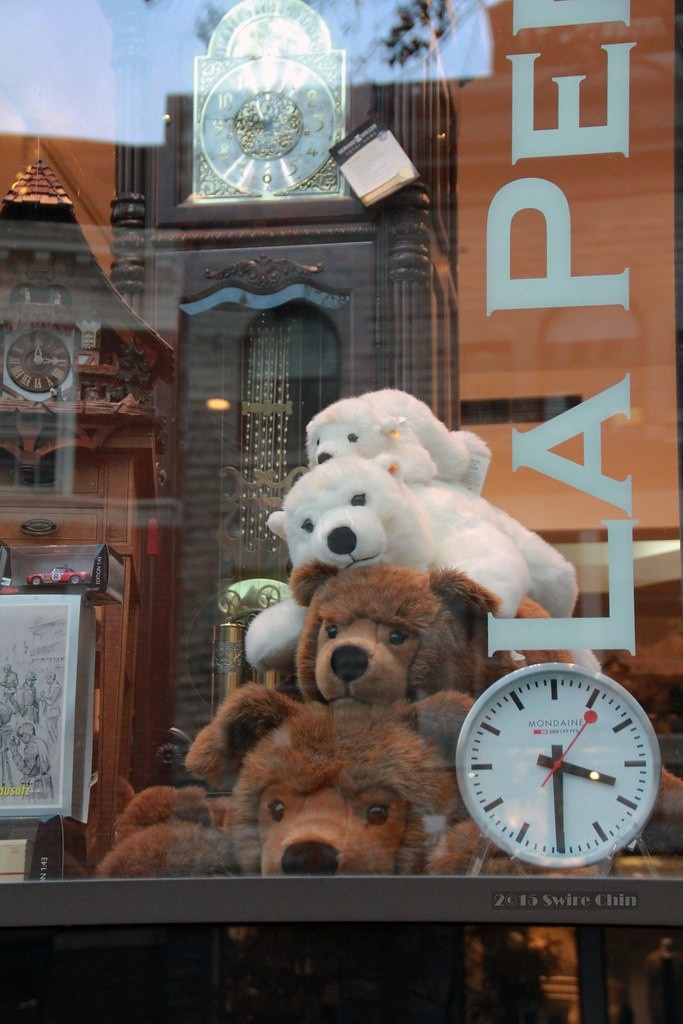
[27,564,92,586]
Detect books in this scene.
[0,593,95,822]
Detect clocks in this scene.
[110,0,459,787]
[453,661,662,881]
[1,160,183,878]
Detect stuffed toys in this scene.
[304,388,490,487]
[91,682,683,883]
[285,560,579,702]
[234,454,604,672]
